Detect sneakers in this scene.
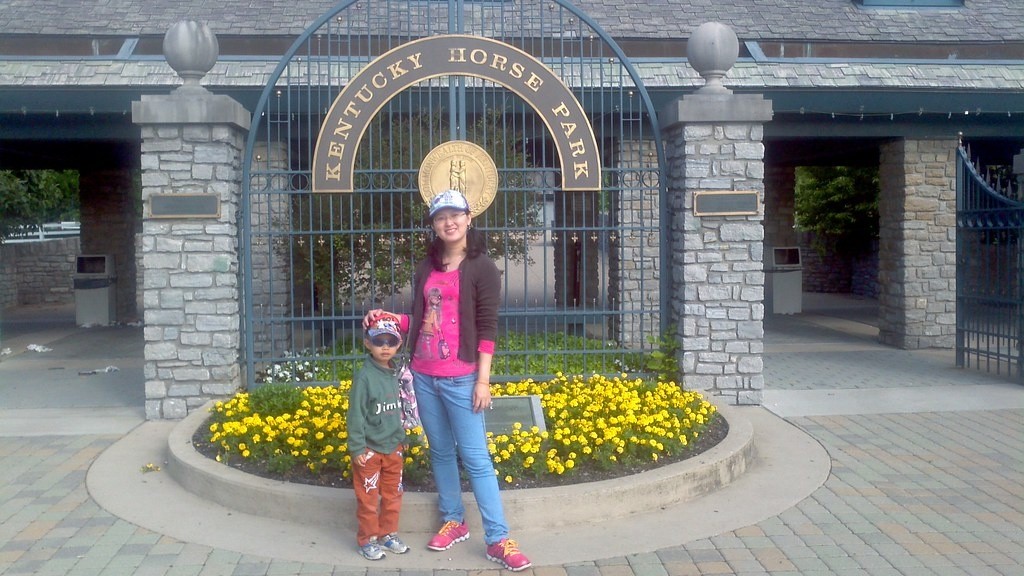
[486,538,531,572]
[427,518,470,551]
[376,532,410,554]
[356,536,387,560]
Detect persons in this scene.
[362,189,531,572]
[346,313,411,560]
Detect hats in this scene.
[367,314,401,340]
[428,190,469,218]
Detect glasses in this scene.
[434,212,466,224]
[371,337,399,347]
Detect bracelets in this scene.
[475,381,489,386]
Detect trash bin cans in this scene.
[763,246,803,315]
[72,254,118,327]
